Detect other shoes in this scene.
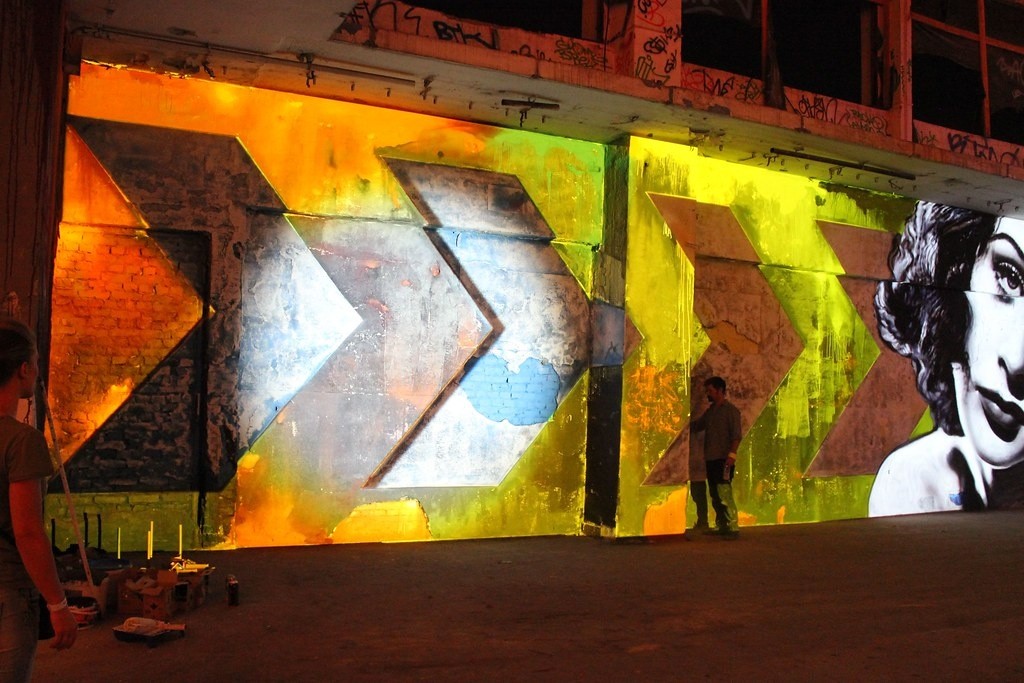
[723,530,739,540]
[703,526,729,534]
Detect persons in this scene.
[0,321,76,683]
[689,378,743,538]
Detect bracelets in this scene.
[44,598,68,613]
